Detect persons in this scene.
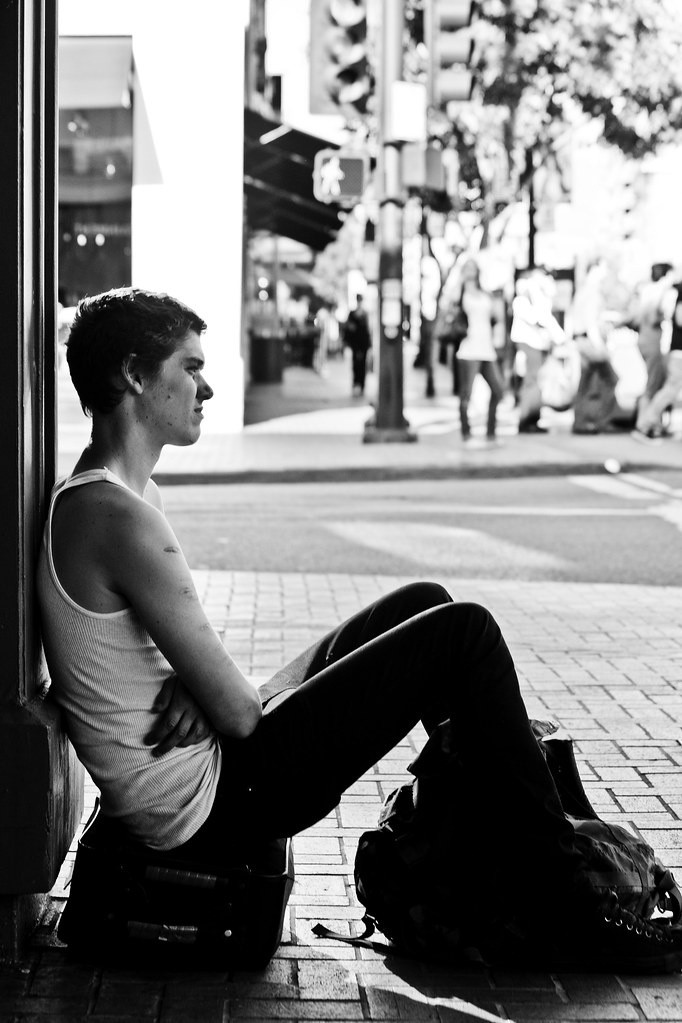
[444,258,682,440]
[311,294,373,396]
[44,288,682,974]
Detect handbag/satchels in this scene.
[354,722,682,979]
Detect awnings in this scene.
[245,107,376,251]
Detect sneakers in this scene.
[518,870,682,975]
